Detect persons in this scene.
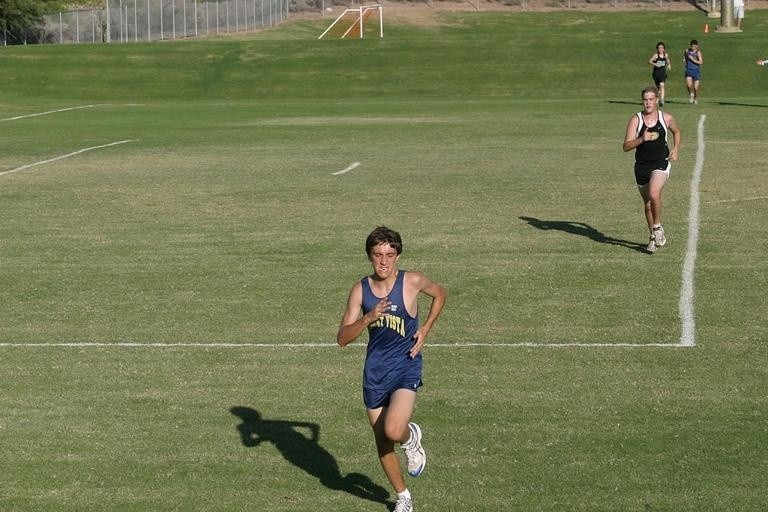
[682,40,703,105]
[622,86,682,254]
[733,1,745,28]
[334,223,447,512]
[647,42,672,109]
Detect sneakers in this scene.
[646,236,656,253]
[651,225,666,247]
[392,495,411,512]
[398,422,426,478]
